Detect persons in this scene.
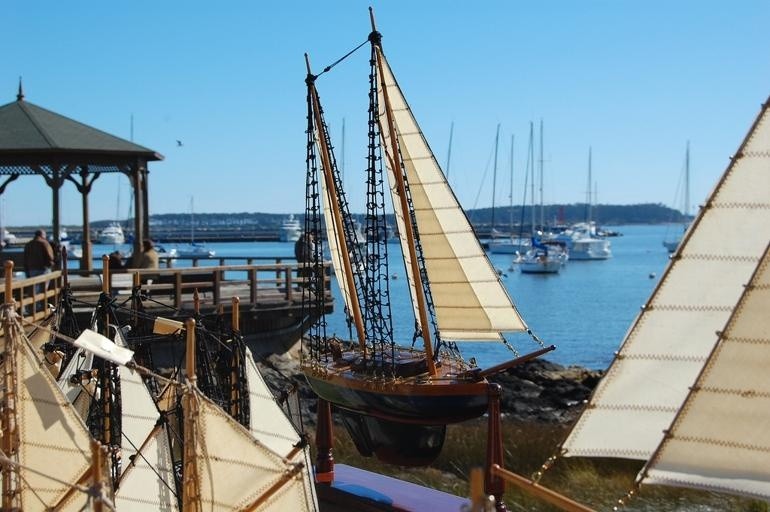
[129,239,160,302]
[110,250,122,269]
[124,254,134,268]
[25,229,54,314]
[296,228,321,291]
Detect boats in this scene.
[126,243,177,264]
[279,213,301,242]
[176,195,215,258]
[97,222,125,245]
[66,247,84,260]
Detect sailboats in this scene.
[460,117,614,272]
[297,2,556,424]
[3,98,769,509]
[660,140,691,253]
